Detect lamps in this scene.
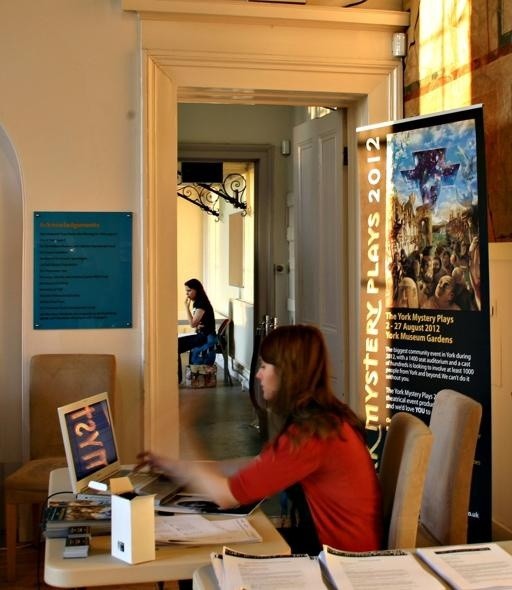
[391,31,408,58]
[281,139,291,156]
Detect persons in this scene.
[178,278,216,389]
[131,323,380,552]
[389,193,481,311]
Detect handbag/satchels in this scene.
[184,363,217,389]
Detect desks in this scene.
[178,312,230,383]
[43,459,291,590]
[191,538,512,590]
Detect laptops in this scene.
[56,392,179,504]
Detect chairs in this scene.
[371,389,484,554]
[4,353,116,583]
[190,317,233,387]
